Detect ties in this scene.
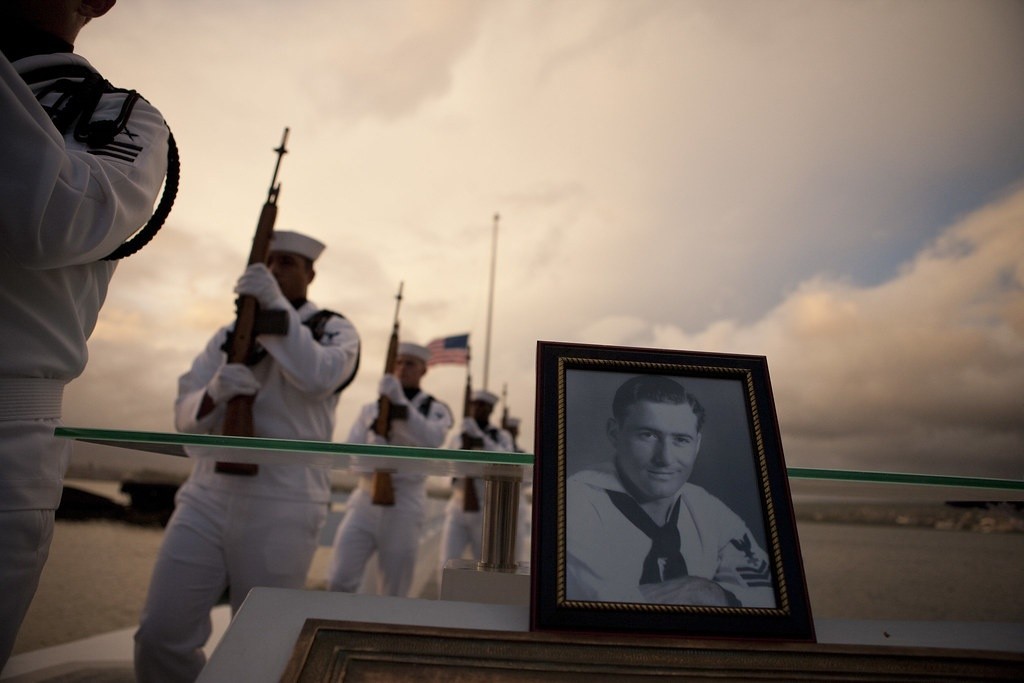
[605,489,689,586]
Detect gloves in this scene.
[462,417,484,438]
[378,374,408,406]
[206,363,261,404]
[234,263,283,305]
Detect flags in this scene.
[426,334,468,367]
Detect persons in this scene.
[566,372,777,608]
[438,390,530,596]
[324,343,452,598]
[0,0,181,668]
[133,231,361,683]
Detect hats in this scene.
[270,231,325,262]
[397,343,431,362]
[471,391,498,405]
[506,419,519,426]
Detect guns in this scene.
[212,124,291,476]
[501,383,510,428]
[370,280,405,507]
[461,345,480,514]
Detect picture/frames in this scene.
[531,340,816,647]
